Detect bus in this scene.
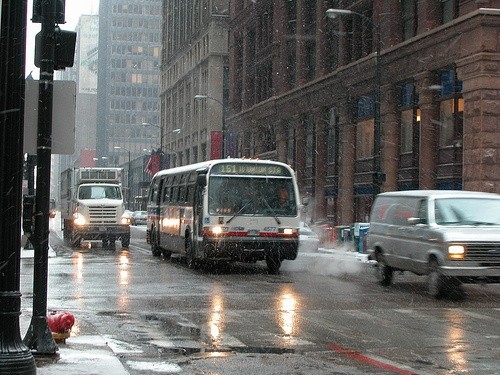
[146,156,309,275]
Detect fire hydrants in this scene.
[48,309,76,345]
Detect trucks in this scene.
[60,166,132,249]
[49,198,57,218]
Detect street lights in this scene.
[114,146,130,210]
[141,121,181,171]
[194,94,226,159]
[325,8,380,194]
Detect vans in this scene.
[365,189,500,301]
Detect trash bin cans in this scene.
[316,223,369,255]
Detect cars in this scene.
[296,220,320,254]
[132,210,148,227]
